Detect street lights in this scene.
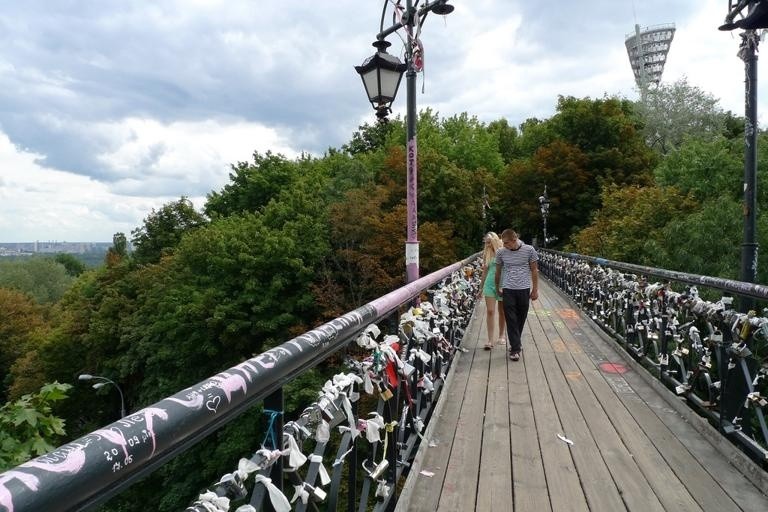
[719,1,767,347]
[354,0,452,306]
[538,185,550,247]
[79,374,126,417]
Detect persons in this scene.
[494,228,539,361]
[475,230,506,352]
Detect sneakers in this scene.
[484,340,495,349]
[498,337,506,345]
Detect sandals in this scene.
[509,344,524,351]
[509,352,520,361]
